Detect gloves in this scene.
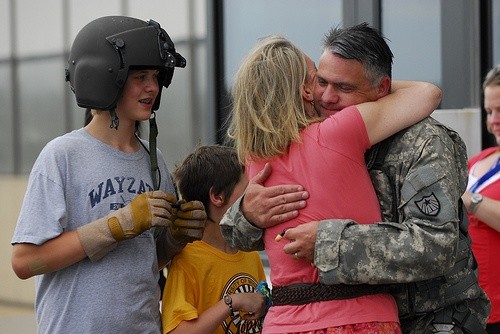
[77,191,177,264]
[156,200,206,271]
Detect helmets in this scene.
[65,16,187,111]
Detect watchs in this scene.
[224,295,234,315]
[468,193,482,213]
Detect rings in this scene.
[295,252,299,260]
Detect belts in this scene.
[272,283,389,305]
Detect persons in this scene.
[161,142,268,334]
[10,15,207,334]
[219,20,492,334]
[461,63,500,334]
[226,36,441,334]
